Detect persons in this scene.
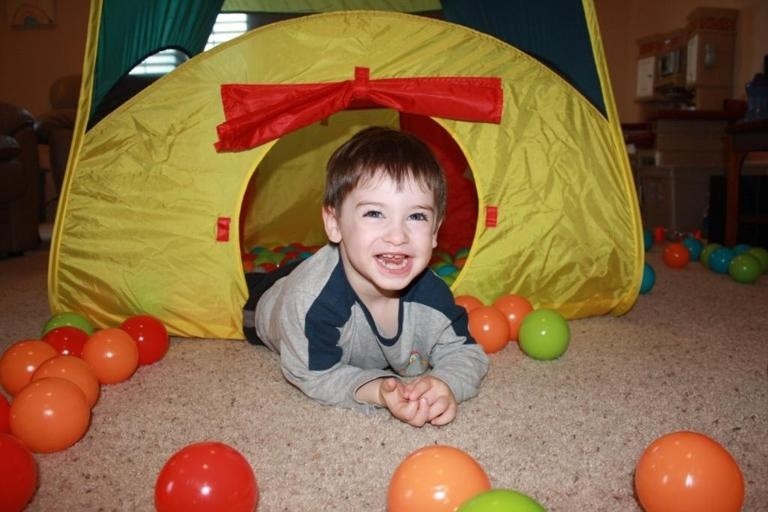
[239,124,496,428]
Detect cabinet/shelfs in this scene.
[620,3,768,247]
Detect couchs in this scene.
[1,72,87,265]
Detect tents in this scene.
[48,0,644,341]
